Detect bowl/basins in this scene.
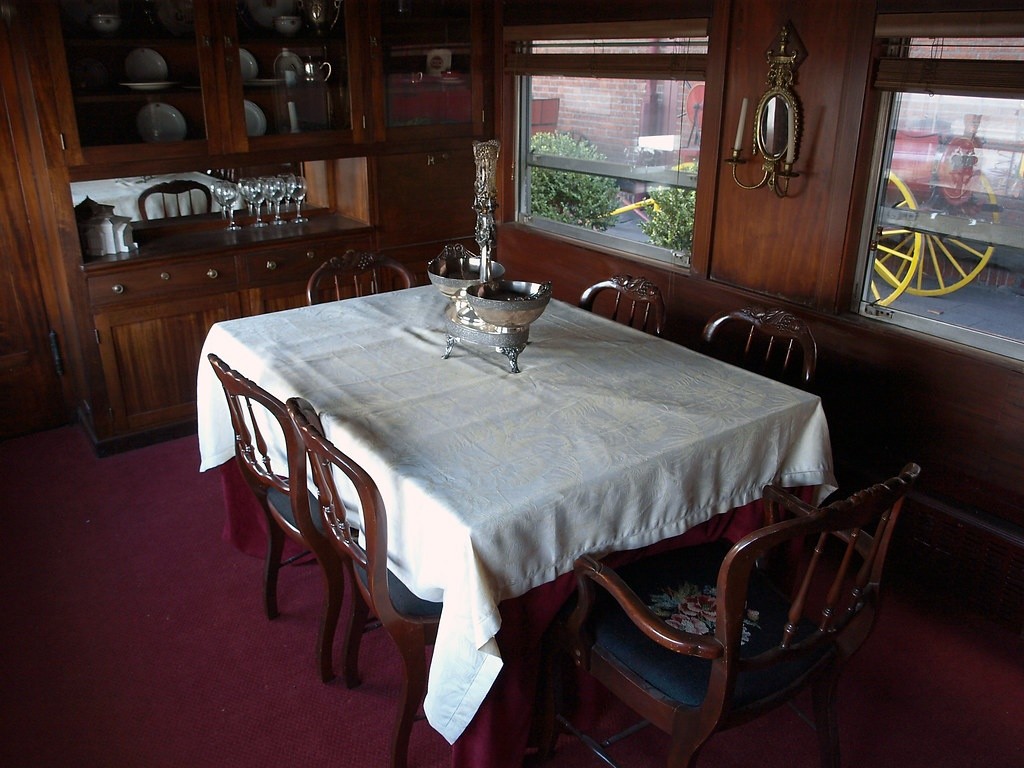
[441,70,459,80]
[271,15,302,36]
[89,15,123,32]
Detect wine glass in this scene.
[238,177,256,217]
[210,181,229,220]
[264,178,287,225]
[277,173,296,212]
[214,182,242,231]
[287,177,309,224]
[244,179,269,228]
[258,175,276,214]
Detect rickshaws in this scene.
[592,75,1003,310]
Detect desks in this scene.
[198,275,838,745]
[68,171,245,222]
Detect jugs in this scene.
[298,55,331,88]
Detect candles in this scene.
[733,92,748,151]
[784,100,798,165]
[288,100,301,134]
[471,141,500,250]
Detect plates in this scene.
[239,48,258,79]
[438,80,465,84]
[141,0,195,38]
[235,0,297,36]
[58,0,133,34]
[119,81,178,91]
[123,48,168,81]
[273,52,304,79]
[243,78,286,87]
[136,102,187,144]
[245,99,266,138]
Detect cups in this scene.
[284,70,298,86]
[426,49,452,78]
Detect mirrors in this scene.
[65,158,331,232]
[753,85,800,162]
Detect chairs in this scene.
[209,353,437,768]
[307,247,411,305]
[579,272,665,337]
[554,465,942,767]
[702,301,819,390]
[138,179,212,223]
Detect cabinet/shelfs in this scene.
[369,144,485,247]
[11,1,367,167]
[370,0,490,141]
[239,226,374,317]
[59,248,239,458]
